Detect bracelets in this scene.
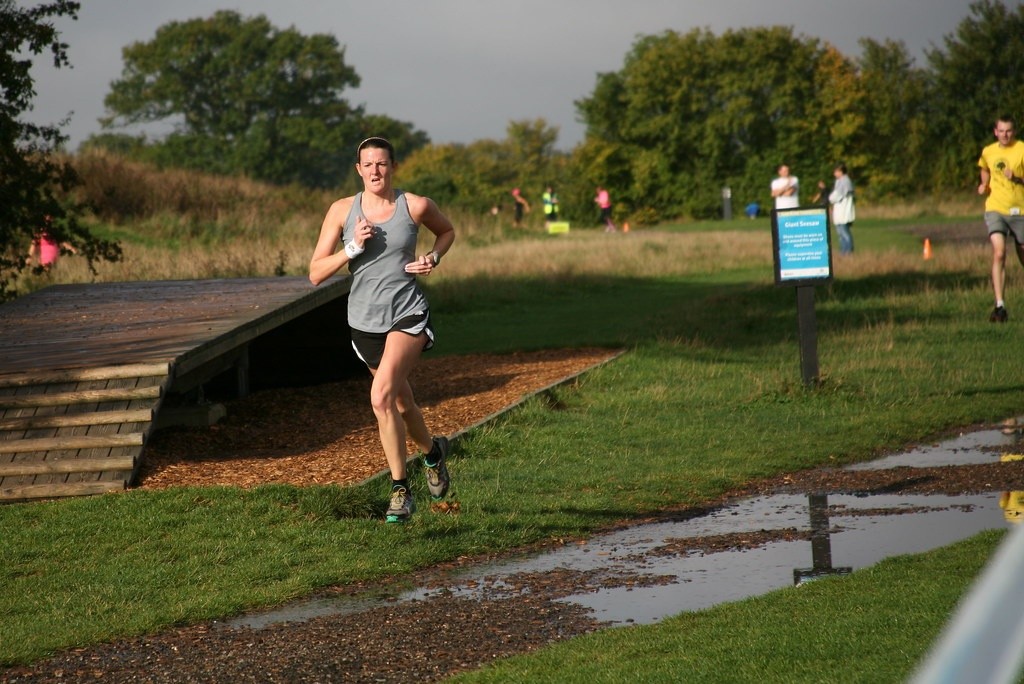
[344,238,364,259]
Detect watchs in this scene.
[429,252,440,268]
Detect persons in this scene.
[491,205,503,218]
[812,180,831,209]
[543,186,560,222]
[829,164,856,255]
[978,115,1024,322]
[512,186,530,229]
[770,164,799,209]
[309,137,456,522]
[24,213,78,272]
[745,201,760,218]
[9,241,22,261]
[595,188,617,233]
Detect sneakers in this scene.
[423,434,449,500]
[385,484,417,523]
[990,305,1008,324]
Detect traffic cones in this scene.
[623,222,630,233]
[921,238,935,262]
